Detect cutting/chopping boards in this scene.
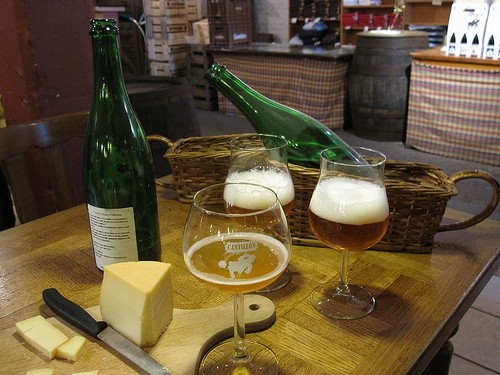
[0,293,279,374]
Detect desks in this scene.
[405,42,500,167]
[0,158,500,375]
[207,41,355,131]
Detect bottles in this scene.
[203,62,380,184]
[459,34,467,56]
[470,35,479,57]
[80,15,164,278]
[486,36,495,60]
[449,33,455,55]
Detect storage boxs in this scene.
[142,0,252,111]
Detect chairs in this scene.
[0,109,91,232]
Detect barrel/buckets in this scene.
[347,30,430,143]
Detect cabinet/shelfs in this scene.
[287,0,454,49]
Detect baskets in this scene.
[265,153,500,254]
[147,134,265,205]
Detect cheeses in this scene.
[99,261,173,348]
[15,315,98,375]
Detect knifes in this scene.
[41,288,175,375]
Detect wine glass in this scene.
[307,146,391,321]
[218,130,296,292]
[181,182,291,375]
